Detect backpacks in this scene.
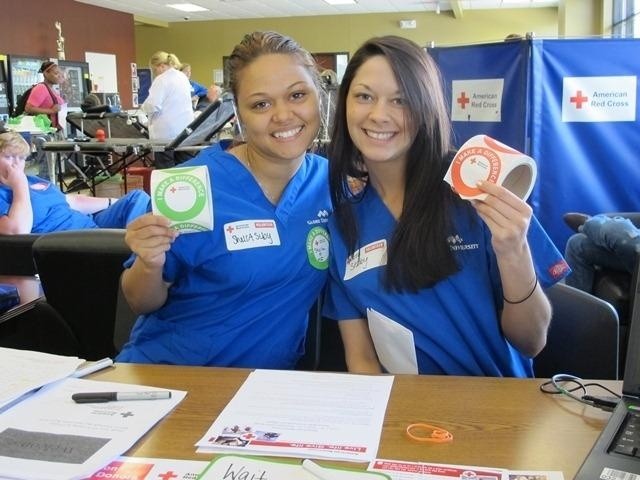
[14,82,58,118]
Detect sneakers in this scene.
[562,211,593,232]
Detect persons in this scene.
[78,92,120,115]
[23,60,67,180]
[317,32,556,380]
[175,59,207,109]
[561,210,640,295]
[0,130,154,238]
[192,85,223,113]
[110,29,354,365]
[135,49,196,170]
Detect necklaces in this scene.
[244,149,277,201]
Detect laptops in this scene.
[573,270,640,480]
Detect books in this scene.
[192,369,398,464]
[1,379,191,478]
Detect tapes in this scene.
[150,165,214,235]
[443,134,537,202]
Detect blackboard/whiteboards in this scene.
[85,51,117,94]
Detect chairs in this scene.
[589,210,639,316]
[114,269,323,372]
[34,232,133,346]
[1,233,44,275]
[532,280,619,380]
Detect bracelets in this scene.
[501,272,538,305]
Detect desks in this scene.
[1,275,45,324]
[41,136,152,196]
[0,347,639,479]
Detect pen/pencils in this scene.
[72,392,171,403]
